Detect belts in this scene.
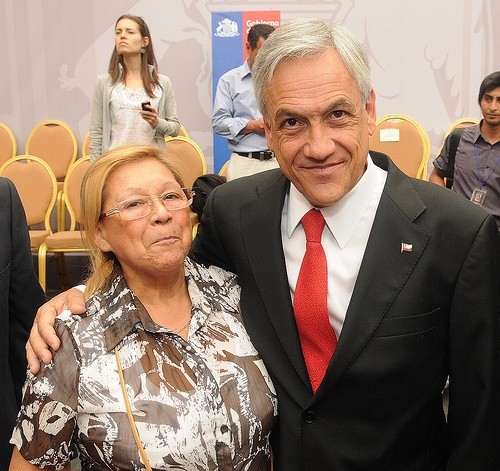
[233,149,275,161]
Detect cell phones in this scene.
[141,101,151,112]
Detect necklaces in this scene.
[173,317,192,338]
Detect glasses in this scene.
[96,187,195,221]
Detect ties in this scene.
[293,208,339,394]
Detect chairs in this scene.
[0,113,483,298]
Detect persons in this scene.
[211,24,281,181]
[88,14,182,166]
[7,145,277,471]
[23,19,500,471]
[430,70,500,232]
[0,175,48,471]
